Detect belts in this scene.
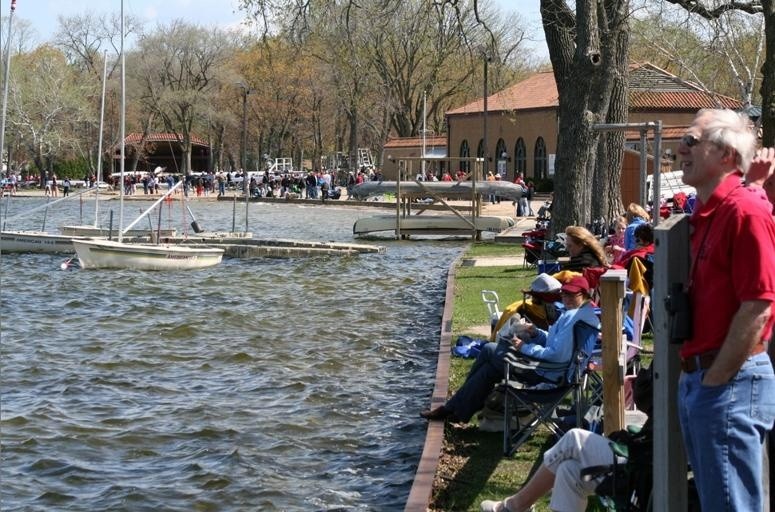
[681,342,765,375]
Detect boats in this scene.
[353,215,514,235]
[347,179,524,201]
[0,224,73,255]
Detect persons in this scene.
[745,144,775,216]
[414,167,697,512]
[0,161,389,201]
[678,107,775,512]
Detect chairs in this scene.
[543,200,554,220]
[522,229,546,270]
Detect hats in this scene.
[532,273,563,292]
[561,276,591,294]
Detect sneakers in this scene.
[481,498,535,511]
[419,406,458,424]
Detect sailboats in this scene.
[71,0,224,269]
[59,48,176,236]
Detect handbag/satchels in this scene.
[477,390,538,433]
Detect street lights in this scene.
[235,80,257,232]
[479,48,492,181]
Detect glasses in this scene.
[683,134,717,146]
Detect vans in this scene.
[93,181,110,188]
